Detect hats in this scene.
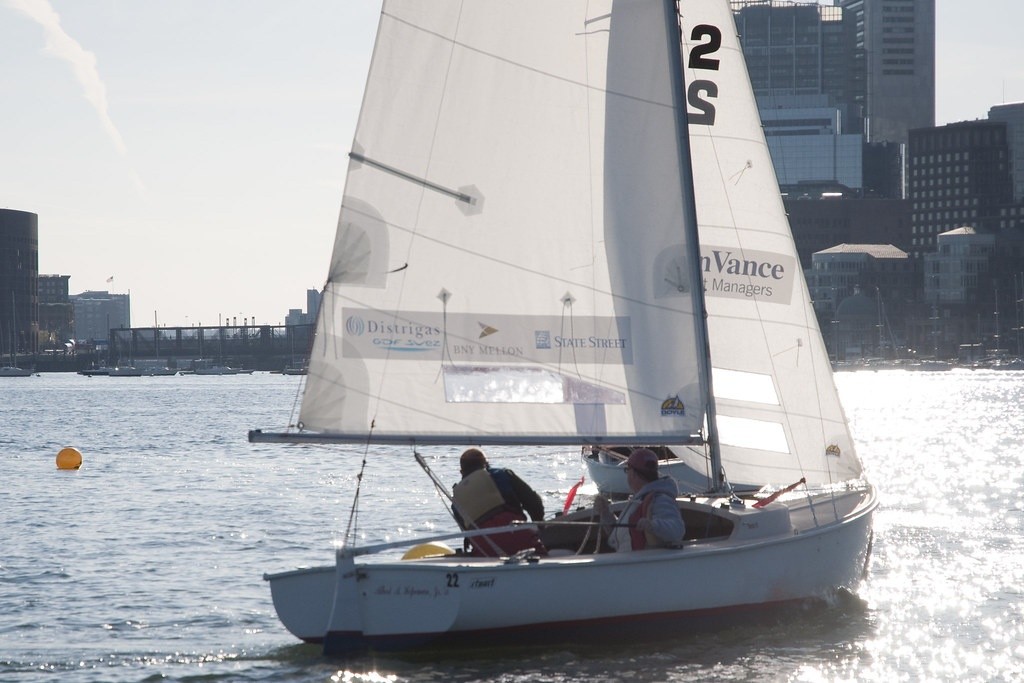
[628,449,658,481]
[460,449,485,471]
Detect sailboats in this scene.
[0,290,37,376]
[142,311,177,376]
[108,336,142,376]
[248,1,876,657]
[77,345,108,375]
[193,313,239,374]
[284,327,305,374]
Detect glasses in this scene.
[624,468,628,473]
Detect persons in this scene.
[450,448,547,558]
[592,447,686,552]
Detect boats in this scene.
[238,370,254,373]
[581,446,762,501]
